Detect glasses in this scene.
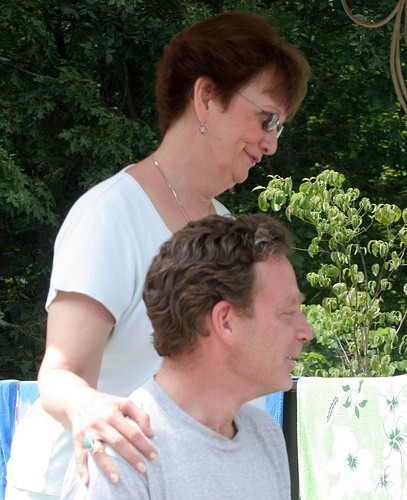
[237,90,284,138]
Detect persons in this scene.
[5,11,311,500]
[60,215,313,500]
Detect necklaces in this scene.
[151,153,212,223]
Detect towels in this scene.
[0,380,18,500]
[297,372,407,500]
[16,378,285,500]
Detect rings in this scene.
[82,434,94,449]
